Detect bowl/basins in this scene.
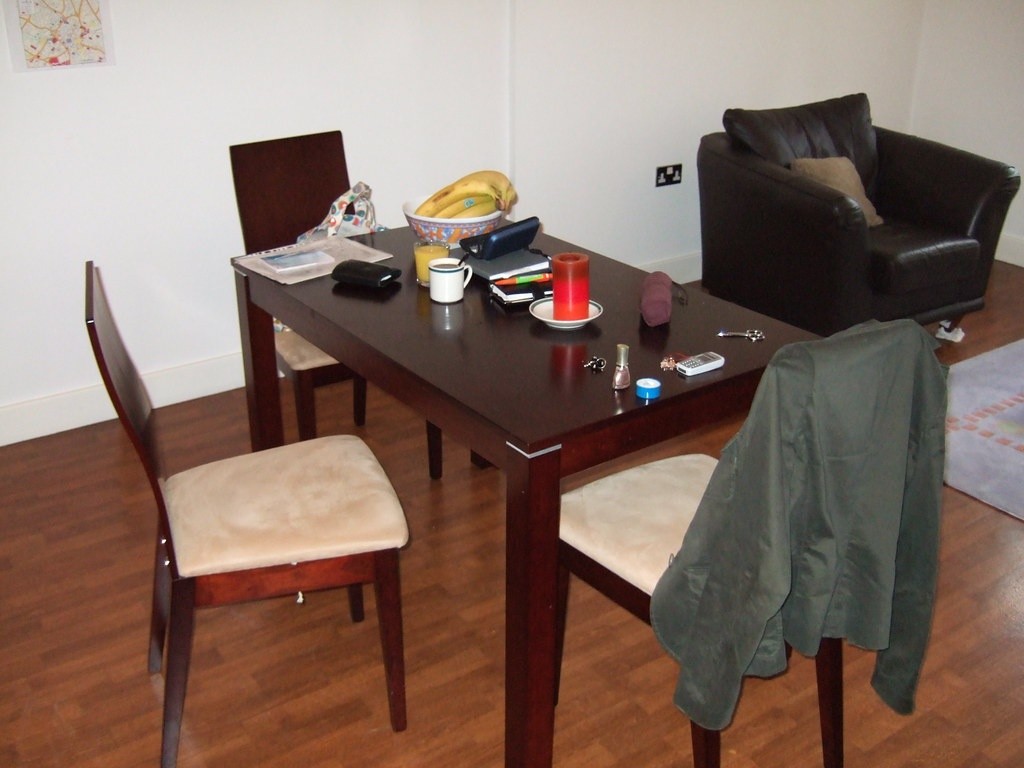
[402,195,503,250]
[528,296,603,332]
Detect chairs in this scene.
[551,319,949,768]
[85,261,411,768]
[230,131,444,481]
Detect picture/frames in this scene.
[3,0,117,73]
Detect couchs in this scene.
[696,93,1020,338]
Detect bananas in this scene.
[415,170,516,219]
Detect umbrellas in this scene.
[641,271,673,326]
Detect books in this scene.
[235,236,393,285]
[449,245,555,305]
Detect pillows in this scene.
[790,156,884,229]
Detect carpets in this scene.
[943,339,1024,521]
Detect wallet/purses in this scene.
[331,259,401,288]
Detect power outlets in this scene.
[656,163,683,187]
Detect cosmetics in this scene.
[612,344,631,389]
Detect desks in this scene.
[231,210,820,768]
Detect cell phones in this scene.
[676,350,726,376]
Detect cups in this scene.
[551,252,590,321]
[428,258,473,304]
[413,240,451,288]
